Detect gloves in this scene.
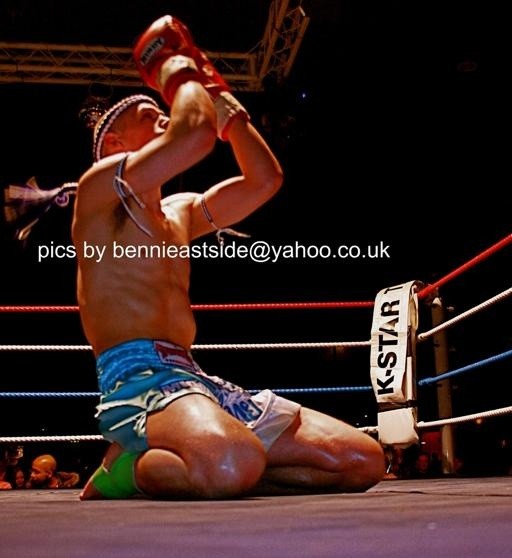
[133,14,249,140]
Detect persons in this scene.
[0,444,79,491]
[70,13,387,497]
[384,444,477,479]
